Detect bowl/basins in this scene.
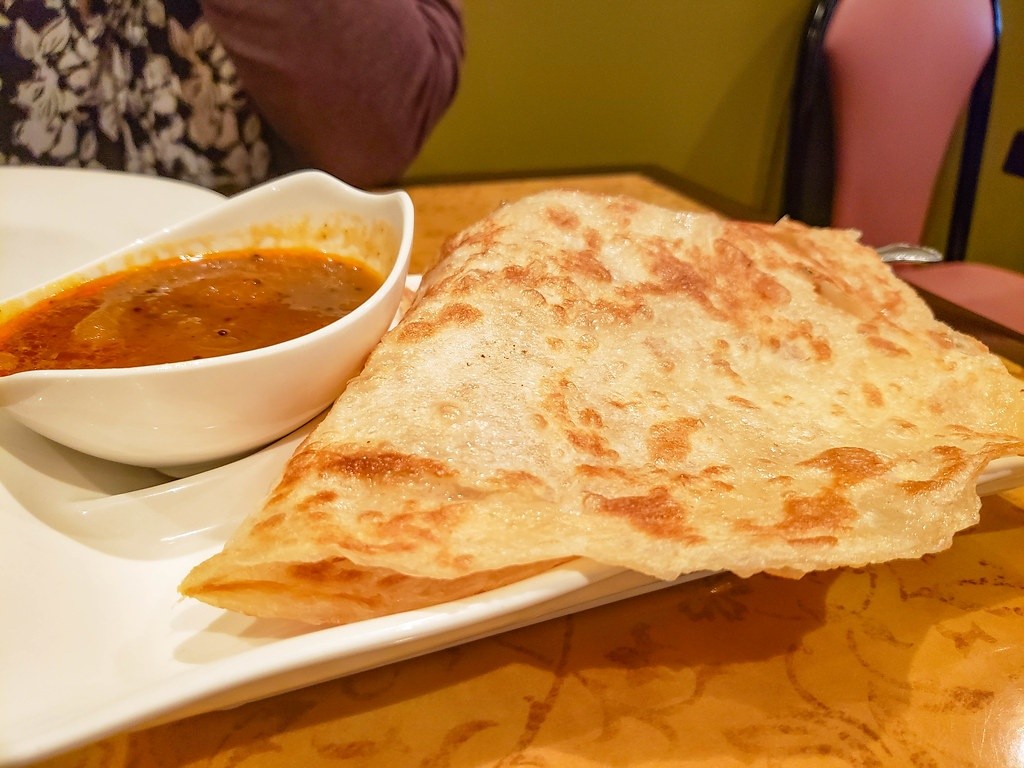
[0,169,415,478]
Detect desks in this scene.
[32,165,1024,767]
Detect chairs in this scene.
[202,1,468,187]
[778,0,1024,339]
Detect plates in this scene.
[0,167,1024,768]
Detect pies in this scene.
[185,191,1024,622]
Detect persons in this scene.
[0,0,465,197]
